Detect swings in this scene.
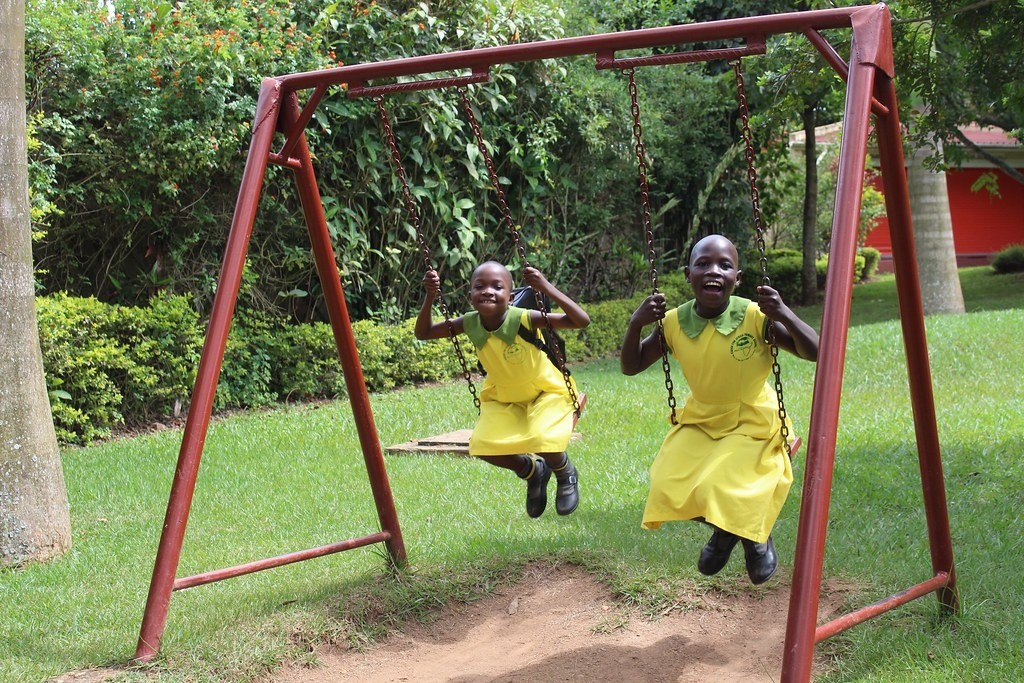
[625,64,803,462]
[371,88,589,432]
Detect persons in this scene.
[621,235,820,585]
[414,261,590,519]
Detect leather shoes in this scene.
[556,466,579,516]
[698,531,740,576]
[741,533,779,585]
[527,459,552,518]
